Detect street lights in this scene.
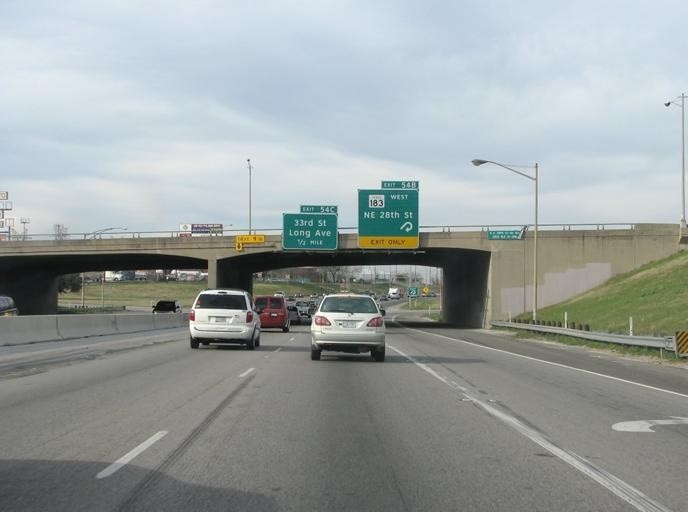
[472,158,538,320]
[664,100,686,219]
[248,157,252,237]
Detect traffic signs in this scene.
[282,213,338,252]
[358,188,420,250]
[301,204,338,215]
[381,182,420,189]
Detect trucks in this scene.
[84,271,125,282]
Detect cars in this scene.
[151,300,181,314]
[159,274,179,280]
[189,287,404,362]
